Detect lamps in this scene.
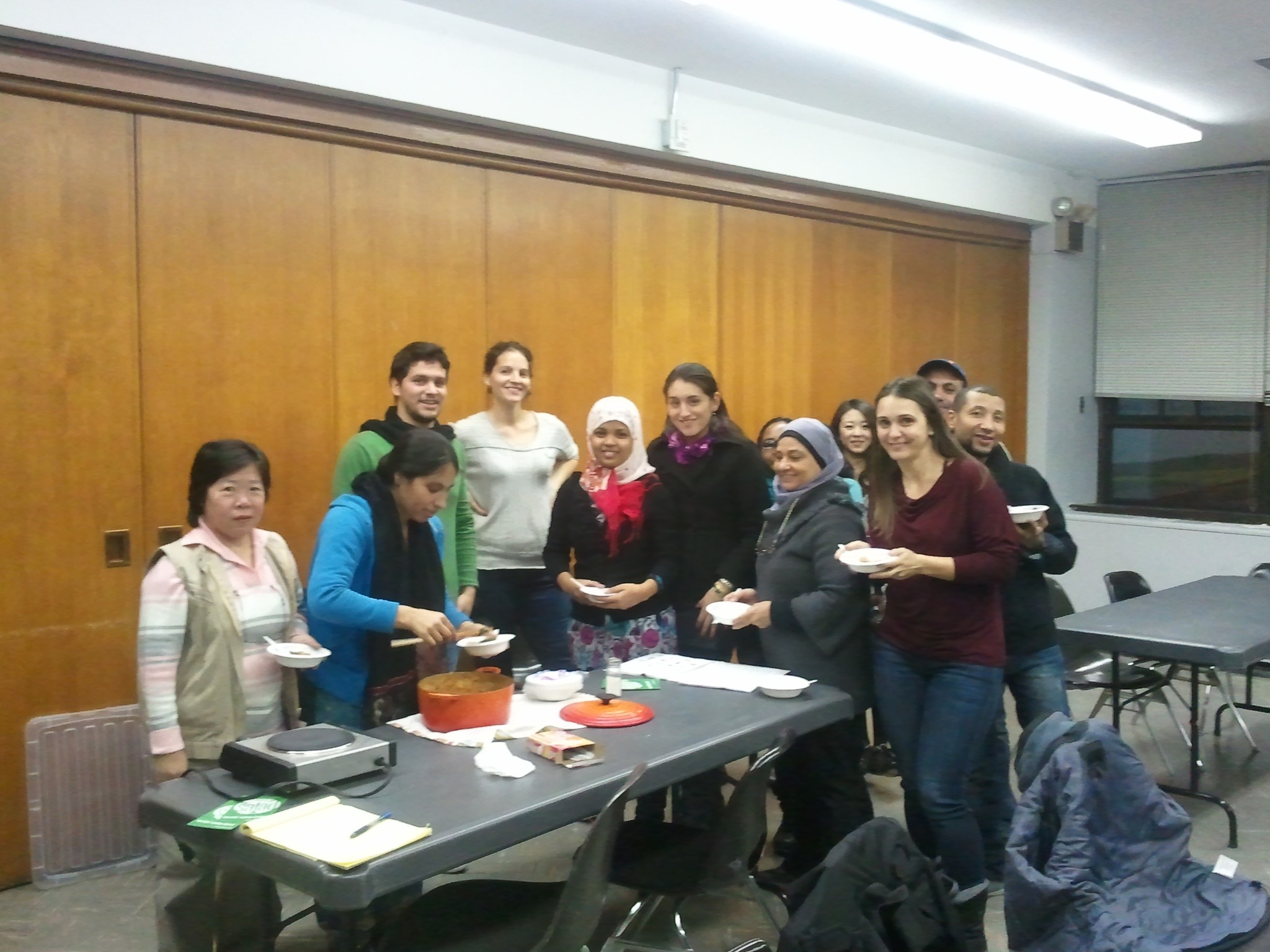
[1052,196,1084,254]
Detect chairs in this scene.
[1044,571,1260,779]
[397,727,793,952]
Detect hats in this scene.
[917,359,967,386]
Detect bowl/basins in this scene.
[1009,504,1050,524]
[758,675,811,698]
[839,547,890,573]
[524,670,584,702]
[266,642,332,668]
[581,587,614,602]
[705,601,752,626]
[455,634,516,658]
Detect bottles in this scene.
[605,657,622,697]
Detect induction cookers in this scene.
[219,722,398,796]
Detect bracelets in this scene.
[720,578,733,591]
[712,582,726,597]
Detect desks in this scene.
[1054,575,1270,849]
[137,664,853,952]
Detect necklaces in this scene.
[755,497,800,556]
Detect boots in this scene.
[953,889,990,952]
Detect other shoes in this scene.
[755,867,795,894]
[771,825,795,856]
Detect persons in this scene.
[307,339,1078,952]
[138,436,321,952]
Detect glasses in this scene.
[759,439,777,450]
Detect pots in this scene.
[419,666,515,733]
